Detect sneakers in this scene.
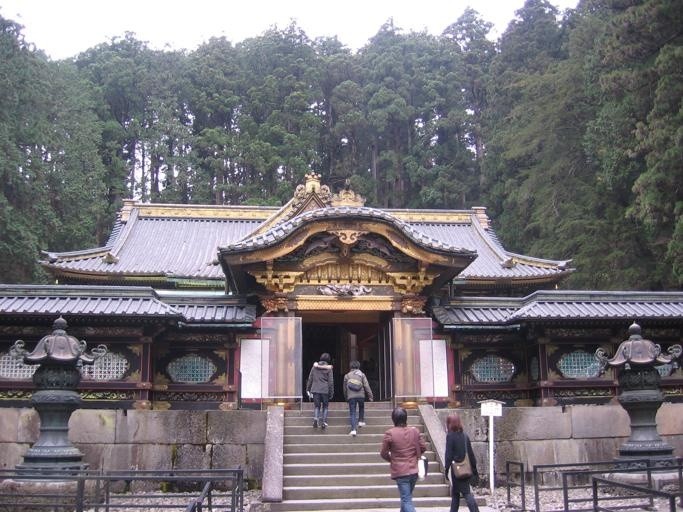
[358,422,365,427]
[417,455,428,482]
[350,430,356,435]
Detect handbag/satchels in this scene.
[452,456,472,479]
[348,380,363,392]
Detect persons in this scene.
[442,412,480,512]
[378,405,427,512]
[340,357,374,437]
[303,351,335,431]
